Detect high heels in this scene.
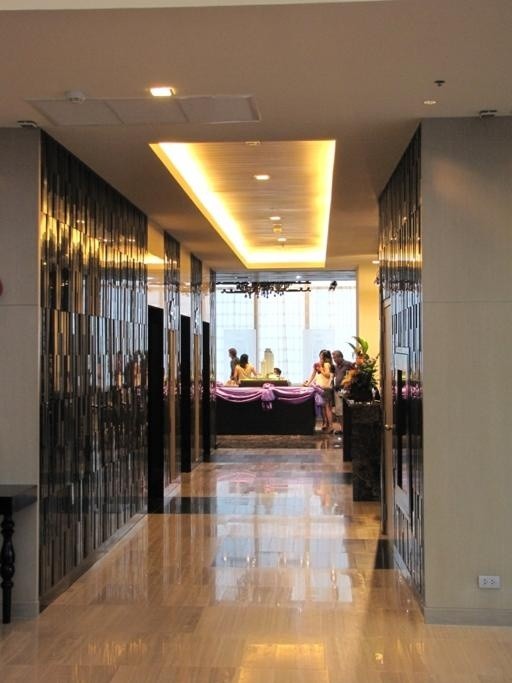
[322,427,336,435]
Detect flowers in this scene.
[348,334,381,403]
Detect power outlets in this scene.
[477,575,501,589]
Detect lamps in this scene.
[236,281,292,299]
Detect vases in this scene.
[353,385,373,402]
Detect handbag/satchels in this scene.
[315,362,335,391]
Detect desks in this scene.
[0,483,37,624]
[239,378,288,387]
[339,394,384,502]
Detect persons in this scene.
[302,348,334,430]
[228,347,240,379]
[316,349,336,434]
[229,353,258,386]
[331,350,353,433]
